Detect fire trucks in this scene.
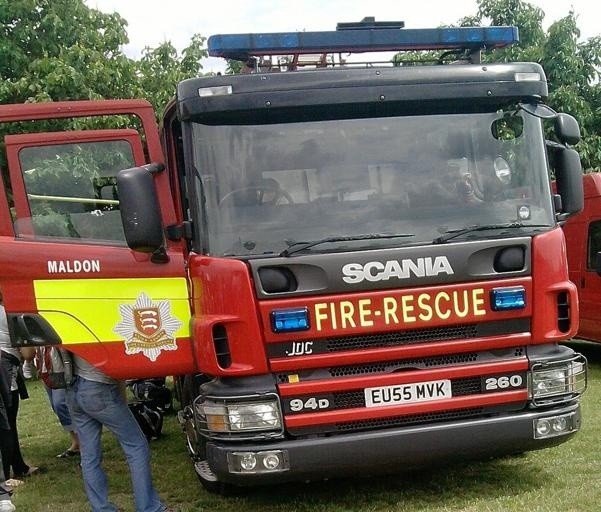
[0,17,588,492]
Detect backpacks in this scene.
[39,347,75,390]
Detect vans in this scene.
[551,172,600,346]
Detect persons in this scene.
[0,295,168,512]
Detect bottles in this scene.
[22,359,33,379]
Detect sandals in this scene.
[55,450,79,459]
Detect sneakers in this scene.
[159,507,181,512]
[21,466,38,478]
[0,500,15,512]
[21,360,31,379]
